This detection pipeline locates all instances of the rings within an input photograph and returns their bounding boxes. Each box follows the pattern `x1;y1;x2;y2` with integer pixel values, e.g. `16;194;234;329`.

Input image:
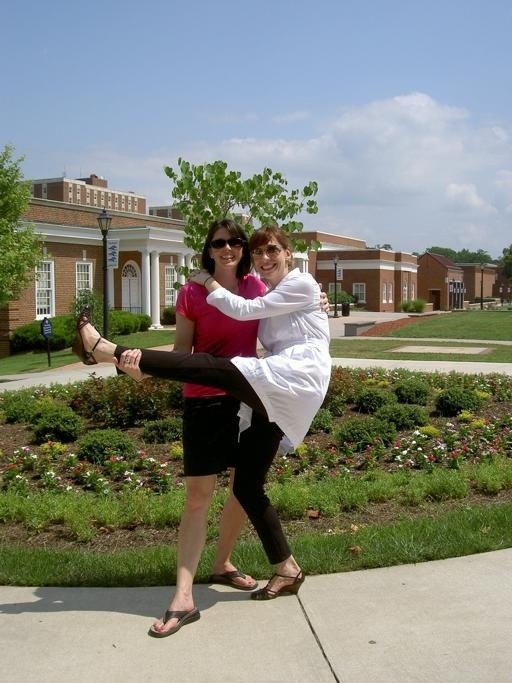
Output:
125;359;130;363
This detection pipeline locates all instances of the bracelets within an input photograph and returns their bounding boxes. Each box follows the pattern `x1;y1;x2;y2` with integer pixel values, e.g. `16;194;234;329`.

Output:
203;275;213;288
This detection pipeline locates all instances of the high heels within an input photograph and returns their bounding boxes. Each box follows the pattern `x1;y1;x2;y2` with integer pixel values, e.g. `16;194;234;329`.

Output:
148;607;200;639
250;569;306;600
211;568;259;591
71;308;103;366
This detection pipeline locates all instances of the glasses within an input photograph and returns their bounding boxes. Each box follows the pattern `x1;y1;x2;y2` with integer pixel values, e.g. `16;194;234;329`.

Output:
253;246;281;257
209;236;243;249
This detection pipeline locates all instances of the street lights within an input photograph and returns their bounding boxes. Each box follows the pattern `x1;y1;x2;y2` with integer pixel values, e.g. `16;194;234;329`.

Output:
96;206;113;340
332;254;340;318
479;261;486;311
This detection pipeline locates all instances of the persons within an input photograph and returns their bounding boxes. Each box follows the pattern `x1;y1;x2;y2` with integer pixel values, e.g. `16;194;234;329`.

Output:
72;224;333;600
113;217;330;638
